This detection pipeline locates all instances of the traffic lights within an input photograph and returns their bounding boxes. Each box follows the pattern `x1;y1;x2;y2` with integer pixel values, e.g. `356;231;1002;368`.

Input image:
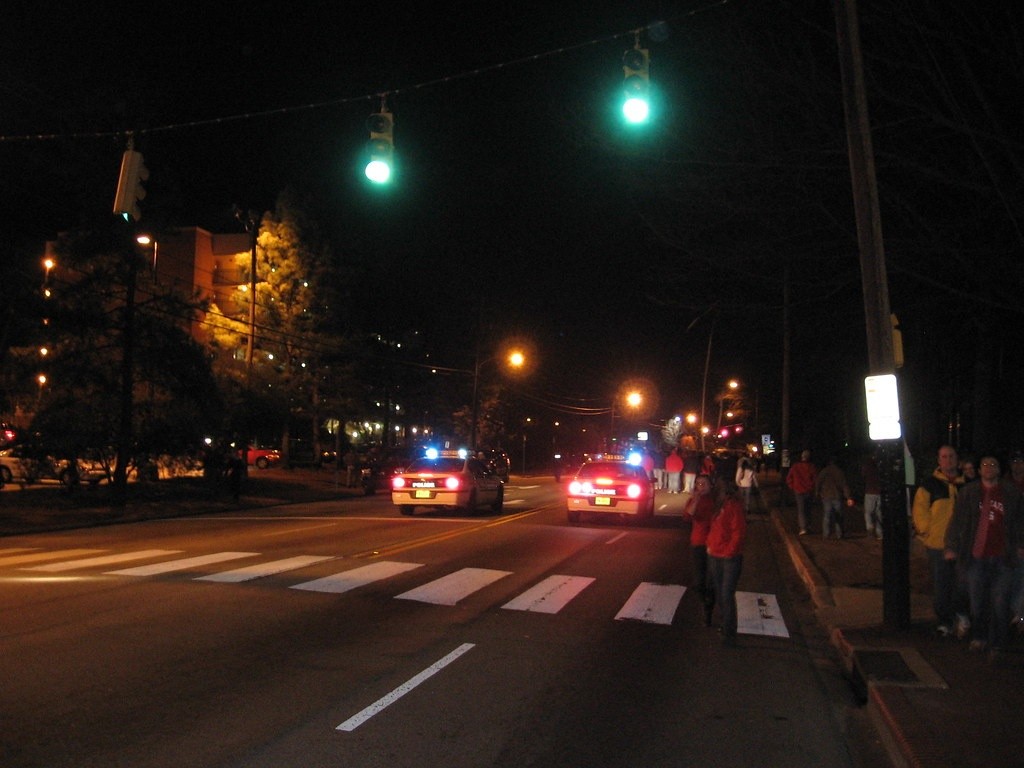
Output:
112;149;150;222
364;111;394;184
620;48;652;125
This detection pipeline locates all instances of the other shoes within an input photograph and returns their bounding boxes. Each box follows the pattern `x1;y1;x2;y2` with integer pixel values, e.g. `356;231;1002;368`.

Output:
970;637;988;653
717;628;744;647
799;528;808;536
937;624;951;636
702;610;712;626
959;615;971;637
989;646;1004;665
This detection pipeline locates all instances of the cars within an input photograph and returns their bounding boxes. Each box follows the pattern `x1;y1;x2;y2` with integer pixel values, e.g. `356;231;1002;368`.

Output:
391;450;506;518
0;445;115;486
361;450;420;495
567;460;660;524
713;448;744;474
199;441;283;469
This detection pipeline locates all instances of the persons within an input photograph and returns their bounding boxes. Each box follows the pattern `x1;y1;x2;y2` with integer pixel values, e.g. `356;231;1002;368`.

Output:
683;475;749;643
642;447;770;512
912;443;1024;663
786;450;882;539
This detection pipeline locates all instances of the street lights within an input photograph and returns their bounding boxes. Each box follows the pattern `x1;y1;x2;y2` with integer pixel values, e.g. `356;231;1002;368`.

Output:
471;346;530;451
